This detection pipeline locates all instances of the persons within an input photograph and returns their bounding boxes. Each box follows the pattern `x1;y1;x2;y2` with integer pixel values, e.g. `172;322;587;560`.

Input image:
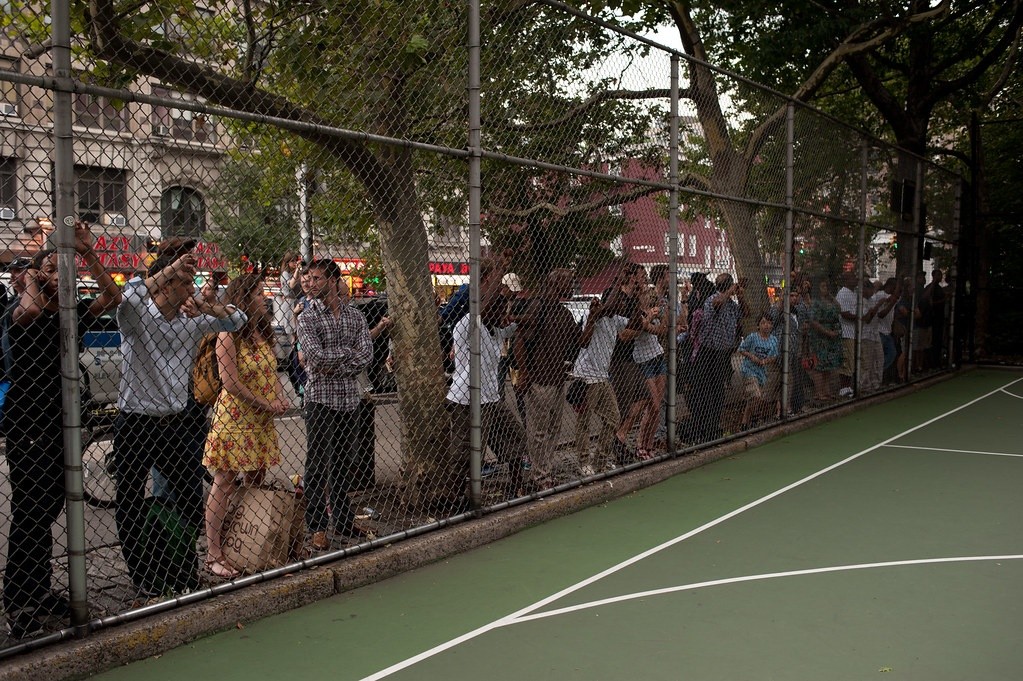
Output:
436;258;969;499
0;220;395;640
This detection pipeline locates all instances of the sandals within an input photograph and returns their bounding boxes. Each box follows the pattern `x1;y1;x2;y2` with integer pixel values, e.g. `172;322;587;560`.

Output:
204;556;241;578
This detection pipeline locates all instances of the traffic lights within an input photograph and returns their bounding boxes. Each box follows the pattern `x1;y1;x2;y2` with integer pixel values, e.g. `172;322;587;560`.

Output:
799;242;804;254
888;237;894;258
893;235;898;256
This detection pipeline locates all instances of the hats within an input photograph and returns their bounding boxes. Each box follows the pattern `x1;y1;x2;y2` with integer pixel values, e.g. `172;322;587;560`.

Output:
917;269;926;275
157;238;197;257
3;258;32;269
690;271;711;283
501;272;524;292
566;380;589;409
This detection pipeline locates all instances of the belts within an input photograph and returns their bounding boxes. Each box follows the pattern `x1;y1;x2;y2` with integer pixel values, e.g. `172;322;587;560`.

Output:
120;405;191;426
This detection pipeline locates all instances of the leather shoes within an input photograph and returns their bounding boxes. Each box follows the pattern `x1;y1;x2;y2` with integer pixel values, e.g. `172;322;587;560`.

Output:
311;531;330;549
333;526;377;538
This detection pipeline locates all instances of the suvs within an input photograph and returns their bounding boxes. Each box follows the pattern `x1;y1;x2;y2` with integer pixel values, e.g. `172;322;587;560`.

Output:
286;294;451;401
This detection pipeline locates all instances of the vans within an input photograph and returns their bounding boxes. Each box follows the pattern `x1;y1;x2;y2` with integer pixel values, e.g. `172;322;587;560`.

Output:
1;273;296;421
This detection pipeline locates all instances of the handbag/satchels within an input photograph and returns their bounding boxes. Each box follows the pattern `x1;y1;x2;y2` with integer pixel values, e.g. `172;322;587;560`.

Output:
261;485;306;559
203;475;295;574
801;333;817;370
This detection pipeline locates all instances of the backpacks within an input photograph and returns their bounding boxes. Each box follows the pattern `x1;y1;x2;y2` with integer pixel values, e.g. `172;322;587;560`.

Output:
193;331;241;405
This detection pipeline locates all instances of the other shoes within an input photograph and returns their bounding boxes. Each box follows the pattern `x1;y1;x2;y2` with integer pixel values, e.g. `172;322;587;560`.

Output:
749;413;760;428
5;606;44;637
814;393;836;400
578;465;595;476
600;462;616;472
739;423;747;431
33;592;67;615
